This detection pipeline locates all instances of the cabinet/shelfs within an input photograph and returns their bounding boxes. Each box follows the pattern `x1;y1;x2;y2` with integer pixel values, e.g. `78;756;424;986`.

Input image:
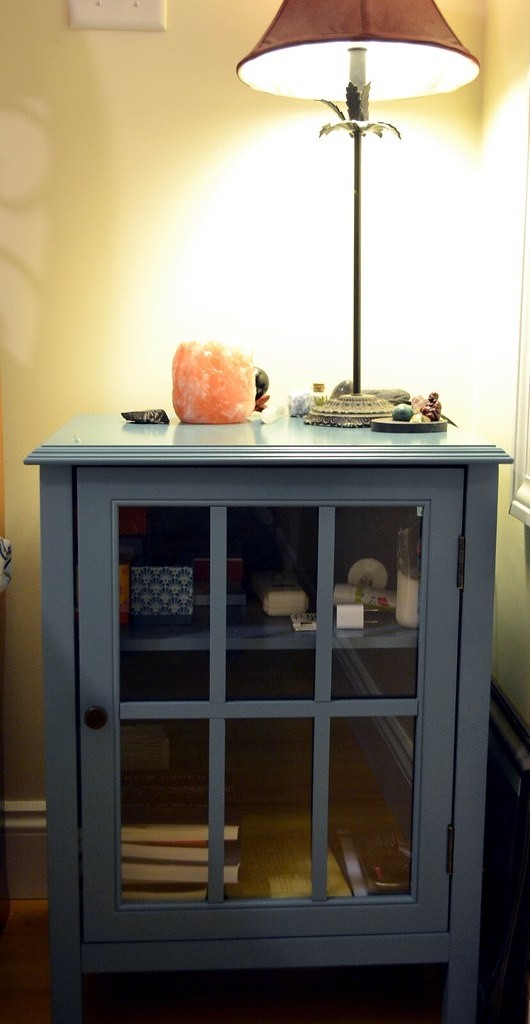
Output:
19;408;513;1023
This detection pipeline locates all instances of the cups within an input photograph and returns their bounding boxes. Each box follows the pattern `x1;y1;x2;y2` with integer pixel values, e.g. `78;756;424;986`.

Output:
395;569;419;629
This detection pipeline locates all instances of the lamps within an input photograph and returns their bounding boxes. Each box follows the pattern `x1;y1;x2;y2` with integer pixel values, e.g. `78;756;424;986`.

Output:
238;0;481;422
